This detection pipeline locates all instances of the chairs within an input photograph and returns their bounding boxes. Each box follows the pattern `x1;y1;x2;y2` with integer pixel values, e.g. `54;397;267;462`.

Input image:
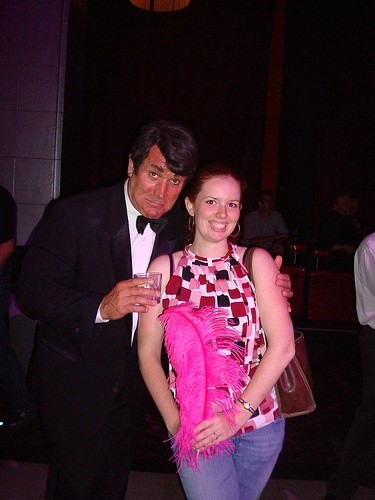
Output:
272;244;358;333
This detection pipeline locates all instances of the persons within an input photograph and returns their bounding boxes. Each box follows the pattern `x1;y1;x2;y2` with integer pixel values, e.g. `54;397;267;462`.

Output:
0;186;40;430
322;233;375;499
317;189;375;248
137;158;295;500
240;186;291;248
15;118;202;500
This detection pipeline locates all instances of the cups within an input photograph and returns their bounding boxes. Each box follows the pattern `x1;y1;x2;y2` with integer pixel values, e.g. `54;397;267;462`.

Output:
134;272;162;305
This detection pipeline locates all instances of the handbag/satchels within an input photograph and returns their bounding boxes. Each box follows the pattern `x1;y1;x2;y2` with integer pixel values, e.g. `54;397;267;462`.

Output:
243;245;318;419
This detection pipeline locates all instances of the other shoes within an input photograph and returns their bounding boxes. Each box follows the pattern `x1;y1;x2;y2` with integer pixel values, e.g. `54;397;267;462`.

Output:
0;406;40;434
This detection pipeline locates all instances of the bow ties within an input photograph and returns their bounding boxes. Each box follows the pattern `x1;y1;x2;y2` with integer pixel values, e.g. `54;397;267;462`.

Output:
135;214;168;234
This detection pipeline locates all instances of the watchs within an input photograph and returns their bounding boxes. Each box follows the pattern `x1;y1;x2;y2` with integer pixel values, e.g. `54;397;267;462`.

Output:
237;396;257;414
